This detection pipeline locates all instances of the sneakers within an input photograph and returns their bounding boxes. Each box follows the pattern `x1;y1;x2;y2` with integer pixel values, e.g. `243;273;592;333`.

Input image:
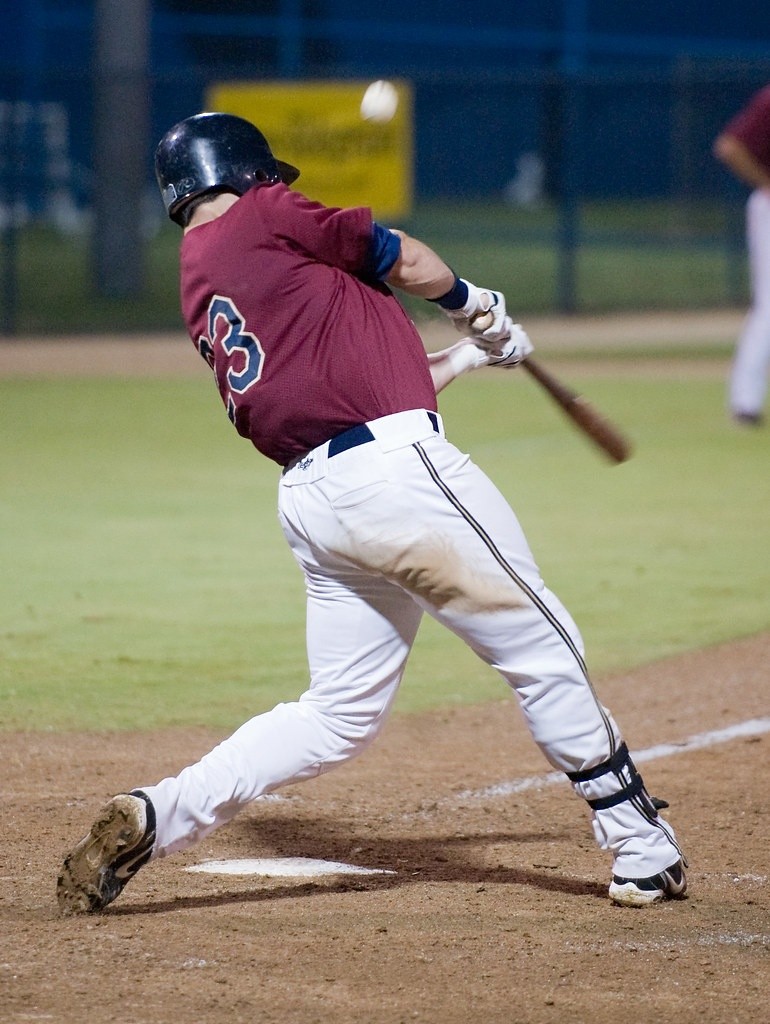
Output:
56;790;156;917
609;855;689;907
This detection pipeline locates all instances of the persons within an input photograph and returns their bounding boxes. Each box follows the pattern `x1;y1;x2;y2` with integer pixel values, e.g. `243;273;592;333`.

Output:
711;83;770;424
56;109;689;917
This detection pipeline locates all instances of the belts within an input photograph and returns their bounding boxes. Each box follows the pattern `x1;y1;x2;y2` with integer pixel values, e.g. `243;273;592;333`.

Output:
328;411;440;458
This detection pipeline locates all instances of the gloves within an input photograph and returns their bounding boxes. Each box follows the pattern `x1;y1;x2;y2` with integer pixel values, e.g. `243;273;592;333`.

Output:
437;278;512;342
458;324;534;373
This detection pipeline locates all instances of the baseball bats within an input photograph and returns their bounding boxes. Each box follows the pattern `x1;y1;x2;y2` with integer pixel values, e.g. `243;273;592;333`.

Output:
471;304;635;466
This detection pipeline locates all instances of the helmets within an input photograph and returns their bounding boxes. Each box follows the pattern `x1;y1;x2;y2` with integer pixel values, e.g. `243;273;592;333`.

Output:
154;112;300;228
